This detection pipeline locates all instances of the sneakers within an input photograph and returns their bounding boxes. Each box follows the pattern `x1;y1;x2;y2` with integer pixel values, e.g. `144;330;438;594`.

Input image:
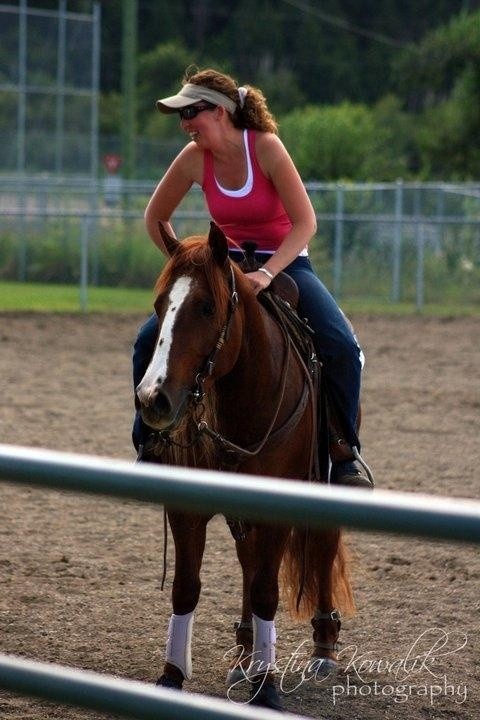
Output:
330;463;371;487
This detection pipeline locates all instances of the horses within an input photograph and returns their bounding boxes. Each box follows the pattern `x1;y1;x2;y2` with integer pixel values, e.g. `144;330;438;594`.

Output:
137;220;355;712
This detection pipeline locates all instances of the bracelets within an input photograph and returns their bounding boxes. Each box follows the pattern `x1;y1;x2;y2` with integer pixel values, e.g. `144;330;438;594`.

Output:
258;268;273;280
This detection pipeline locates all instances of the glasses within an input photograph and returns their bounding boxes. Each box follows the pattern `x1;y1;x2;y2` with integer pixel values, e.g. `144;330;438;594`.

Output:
179;106;212;120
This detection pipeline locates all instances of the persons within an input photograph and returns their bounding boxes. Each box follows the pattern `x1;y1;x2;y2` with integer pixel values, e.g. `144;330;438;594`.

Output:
132;65;373;487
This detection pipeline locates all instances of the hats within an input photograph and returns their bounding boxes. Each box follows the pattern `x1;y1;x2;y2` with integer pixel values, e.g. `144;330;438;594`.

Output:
156;83;238;115
